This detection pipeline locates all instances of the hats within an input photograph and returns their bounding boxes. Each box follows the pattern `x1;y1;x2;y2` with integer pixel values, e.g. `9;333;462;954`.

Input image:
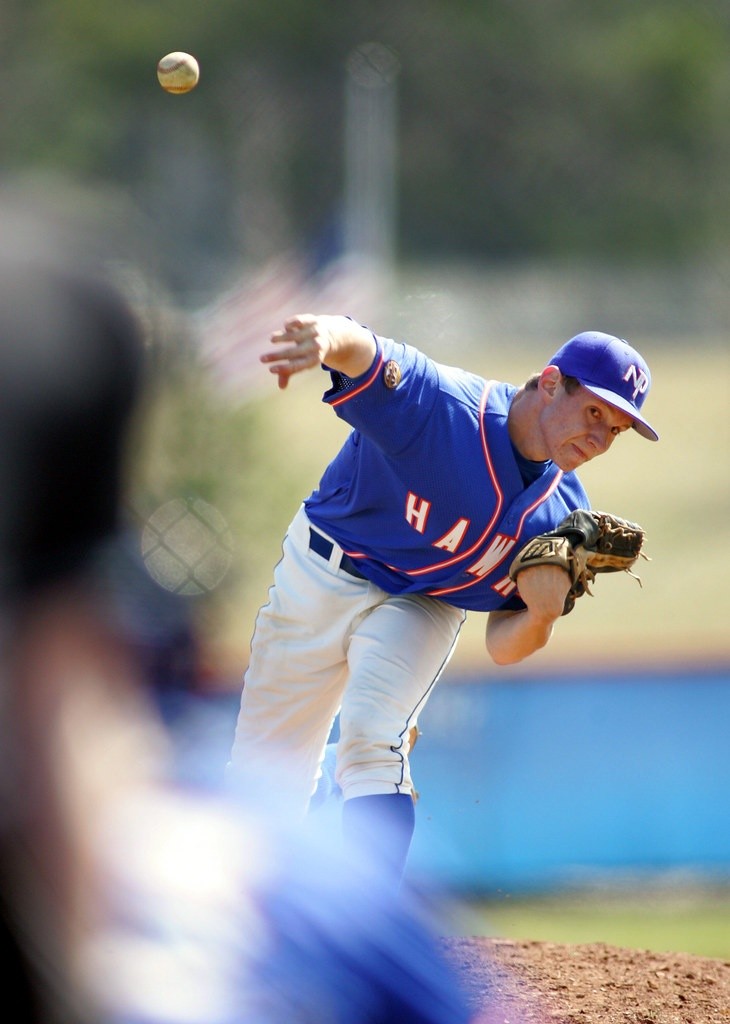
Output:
549;332;660;448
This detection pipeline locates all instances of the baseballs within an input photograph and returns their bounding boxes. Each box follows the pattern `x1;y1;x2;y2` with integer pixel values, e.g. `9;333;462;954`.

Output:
157;52;200;95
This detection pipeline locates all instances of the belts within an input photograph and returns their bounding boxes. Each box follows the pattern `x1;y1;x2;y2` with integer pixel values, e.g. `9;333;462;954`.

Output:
306;528;370;582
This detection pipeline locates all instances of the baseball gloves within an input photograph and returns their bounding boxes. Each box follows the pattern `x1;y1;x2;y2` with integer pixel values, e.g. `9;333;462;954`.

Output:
509;509;653;616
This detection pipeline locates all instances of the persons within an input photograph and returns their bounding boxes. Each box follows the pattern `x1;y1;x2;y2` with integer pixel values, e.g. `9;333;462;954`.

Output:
231;314;659;896
0;238;476;1024
